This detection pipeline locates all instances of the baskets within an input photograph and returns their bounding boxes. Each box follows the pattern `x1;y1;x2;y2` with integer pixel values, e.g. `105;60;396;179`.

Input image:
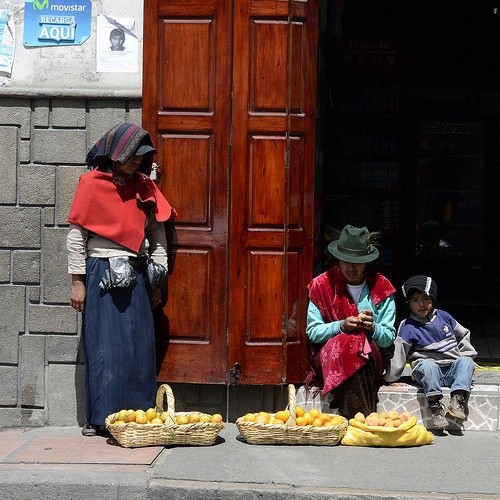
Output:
105;384;224;448
236;383;349;446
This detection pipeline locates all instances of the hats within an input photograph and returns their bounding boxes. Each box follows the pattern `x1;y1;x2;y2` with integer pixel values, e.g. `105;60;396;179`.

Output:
401;275;438;305
132;145;157;158
327;224;380;263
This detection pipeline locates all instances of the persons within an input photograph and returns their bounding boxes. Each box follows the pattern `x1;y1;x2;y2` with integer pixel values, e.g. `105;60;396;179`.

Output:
305;224;396;420
67;122;179;436
381;274;478;429
108;28;126;51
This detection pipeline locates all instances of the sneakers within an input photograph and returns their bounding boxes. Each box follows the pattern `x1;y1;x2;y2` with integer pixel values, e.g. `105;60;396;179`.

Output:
445;391;469;421
426;398;449;429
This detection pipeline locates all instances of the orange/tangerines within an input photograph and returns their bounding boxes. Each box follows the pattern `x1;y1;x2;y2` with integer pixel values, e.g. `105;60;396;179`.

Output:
111;408;223;424
243;406;345;428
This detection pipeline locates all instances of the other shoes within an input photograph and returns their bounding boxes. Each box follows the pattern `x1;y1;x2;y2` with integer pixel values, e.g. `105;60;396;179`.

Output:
82;421;100;435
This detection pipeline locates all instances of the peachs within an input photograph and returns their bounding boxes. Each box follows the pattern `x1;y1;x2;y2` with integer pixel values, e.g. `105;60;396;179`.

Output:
355;411;410;429
